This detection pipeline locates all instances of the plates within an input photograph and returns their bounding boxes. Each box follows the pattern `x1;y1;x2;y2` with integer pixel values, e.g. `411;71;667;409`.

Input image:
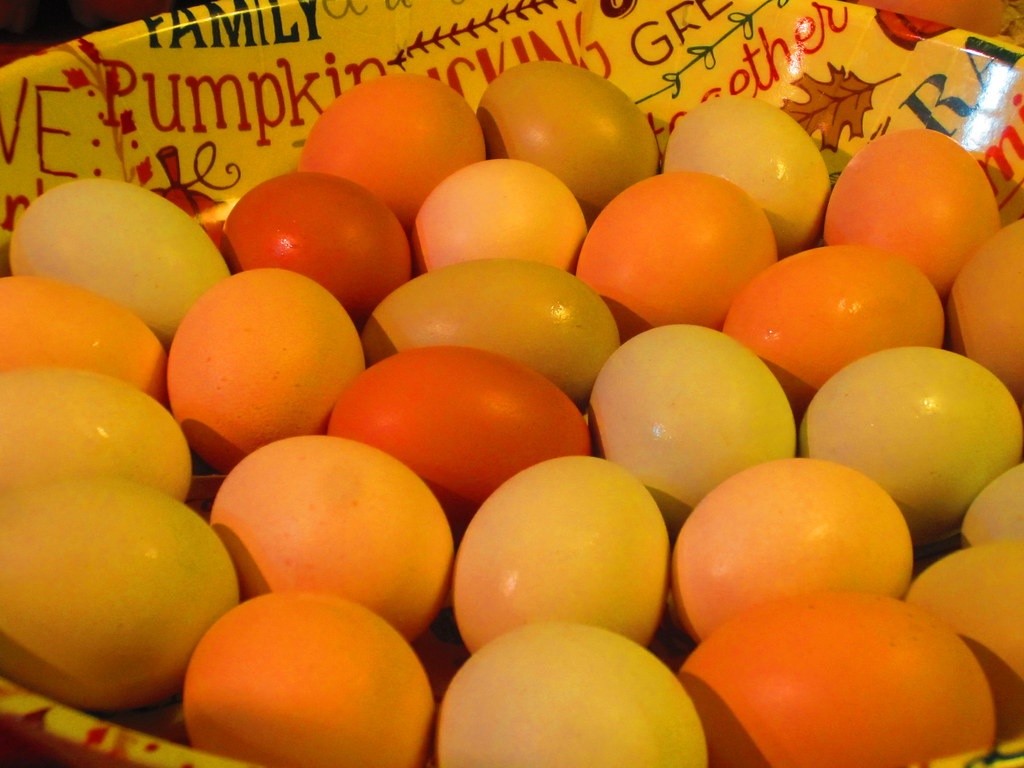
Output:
0;0;1024;768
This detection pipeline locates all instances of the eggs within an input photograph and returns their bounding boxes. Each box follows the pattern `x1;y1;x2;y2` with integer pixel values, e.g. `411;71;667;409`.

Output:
0;58;1022;768
